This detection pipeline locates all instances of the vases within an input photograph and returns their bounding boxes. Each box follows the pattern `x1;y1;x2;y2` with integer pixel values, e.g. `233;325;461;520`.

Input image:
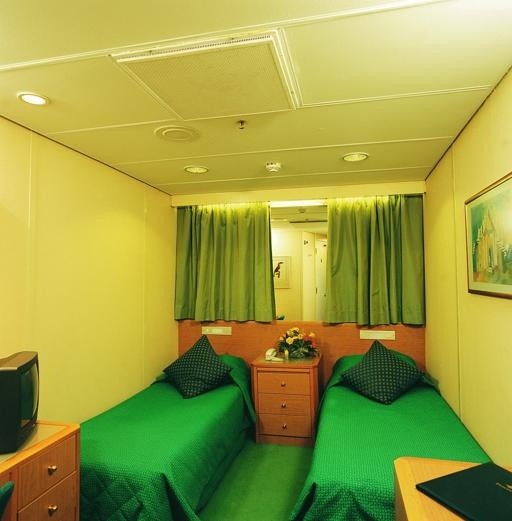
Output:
287;350;304;358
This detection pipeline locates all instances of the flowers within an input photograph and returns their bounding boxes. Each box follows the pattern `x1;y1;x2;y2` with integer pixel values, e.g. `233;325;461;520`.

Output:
274;327;320;360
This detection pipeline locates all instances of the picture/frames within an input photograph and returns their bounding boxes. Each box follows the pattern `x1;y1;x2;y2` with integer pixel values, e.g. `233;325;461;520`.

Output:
462;171;512;301
272;255;290;289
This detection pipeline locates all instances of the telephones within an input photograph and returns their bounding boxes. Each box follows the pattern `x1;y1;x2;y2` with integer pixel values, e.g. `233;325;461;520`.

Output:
265;347;277;361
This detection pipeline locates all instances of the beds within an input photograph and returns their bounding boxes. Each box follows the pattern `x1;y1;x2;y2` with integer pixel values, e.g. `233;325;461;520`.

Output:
285;348;493;521
80;353;257;521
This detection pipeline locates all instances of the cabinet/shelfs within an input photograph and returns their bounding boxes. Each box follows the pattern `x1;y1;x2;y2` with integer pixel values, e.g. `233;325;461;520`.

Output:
391;454;512;521
0;418;82;521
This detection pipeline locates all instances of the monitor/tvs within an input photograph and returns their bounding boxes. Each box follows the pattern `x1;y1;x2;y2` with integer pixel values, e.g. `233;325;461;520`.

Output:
0;350;41;454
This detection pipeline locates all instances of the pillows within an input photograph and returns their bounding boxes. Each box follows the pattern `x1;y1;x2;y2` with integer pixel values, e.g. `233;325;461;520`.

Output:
339;339;425;406
161;334;234;400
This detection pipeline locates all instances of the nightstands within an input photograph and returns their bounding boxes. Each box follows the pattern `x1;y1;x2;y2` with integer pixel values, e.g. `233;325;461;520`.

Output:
249;352;325;449
